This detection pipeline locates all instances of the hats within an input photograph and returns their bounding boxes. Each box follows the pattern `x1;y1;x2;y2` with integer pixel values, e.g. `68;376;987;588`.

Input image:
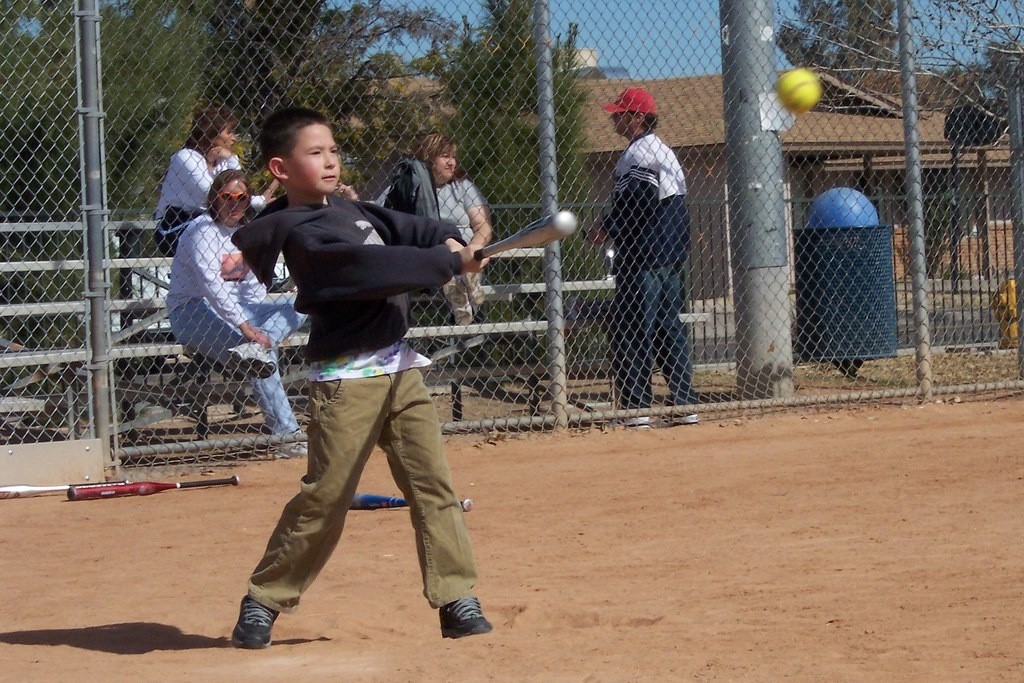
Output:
605;87;656;113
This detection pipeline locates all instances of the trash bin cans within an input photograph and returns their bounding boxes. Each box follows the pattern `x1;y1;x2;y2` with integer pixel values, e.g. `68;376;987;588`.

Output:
791;222;901;376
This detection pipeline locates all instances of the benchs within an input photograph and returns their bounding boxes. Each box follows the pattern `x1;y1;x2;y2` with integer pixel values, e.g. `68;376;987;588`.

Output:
131;247;710;441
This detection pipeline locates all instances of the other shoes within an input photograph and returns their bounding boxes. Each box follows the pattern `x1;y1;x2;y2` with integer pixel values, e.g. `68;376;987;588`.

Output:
610;412;650;427
461;271;484;304
442;276;472;325
662;410;699;423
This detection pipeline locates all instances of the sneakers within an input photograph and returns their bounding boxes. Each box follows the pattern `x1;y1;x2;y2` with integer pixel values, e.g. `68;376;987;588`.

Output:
439;594;492;639
227;341;276;379
231;594;281;649
273;429;309;459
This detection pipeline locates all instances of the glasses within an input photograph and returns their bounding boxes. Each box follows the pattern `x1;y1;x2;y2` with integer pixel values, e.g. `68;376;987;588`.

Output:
218;192;249;204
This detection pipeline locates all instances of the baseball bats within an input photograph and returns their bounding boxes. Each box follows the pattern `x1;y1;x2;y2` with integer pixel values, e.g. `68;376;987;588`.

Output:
349;492;473;511
68;475;240;501
0;480;134;499
474;210;578;261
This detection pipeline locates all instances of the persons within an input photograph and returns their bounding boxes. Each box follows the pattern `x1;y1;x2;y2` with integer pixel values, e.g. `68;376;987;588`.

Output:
231;108;493;652
151;101;493;458
587;86;702;430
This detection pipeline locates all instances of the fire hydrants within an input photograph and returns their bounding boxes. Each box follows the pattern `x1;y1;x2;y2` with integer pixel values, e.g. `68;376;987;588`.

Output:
991;277;1019;349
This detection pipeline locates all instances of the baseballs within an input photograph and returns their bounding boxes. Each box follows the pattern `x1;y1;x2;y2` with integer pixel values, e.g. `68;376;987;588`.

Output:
776;68;822;111
